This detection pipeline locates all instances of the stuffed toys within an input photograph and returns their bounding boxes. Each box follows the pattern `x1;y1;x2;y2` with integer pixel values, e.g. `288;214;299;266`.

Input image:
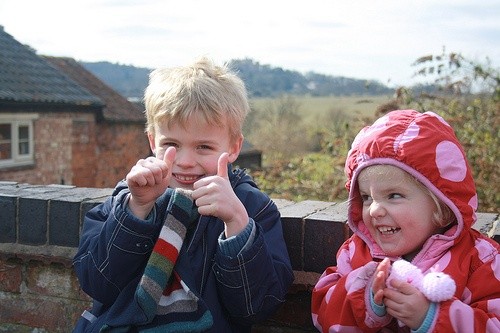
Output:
386;259;456;328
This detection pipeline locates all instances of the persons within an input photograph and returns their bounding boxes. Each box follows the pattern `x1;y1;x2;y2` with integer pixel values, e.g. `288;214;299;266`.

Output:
311;109;500;333
71;55;295;333
